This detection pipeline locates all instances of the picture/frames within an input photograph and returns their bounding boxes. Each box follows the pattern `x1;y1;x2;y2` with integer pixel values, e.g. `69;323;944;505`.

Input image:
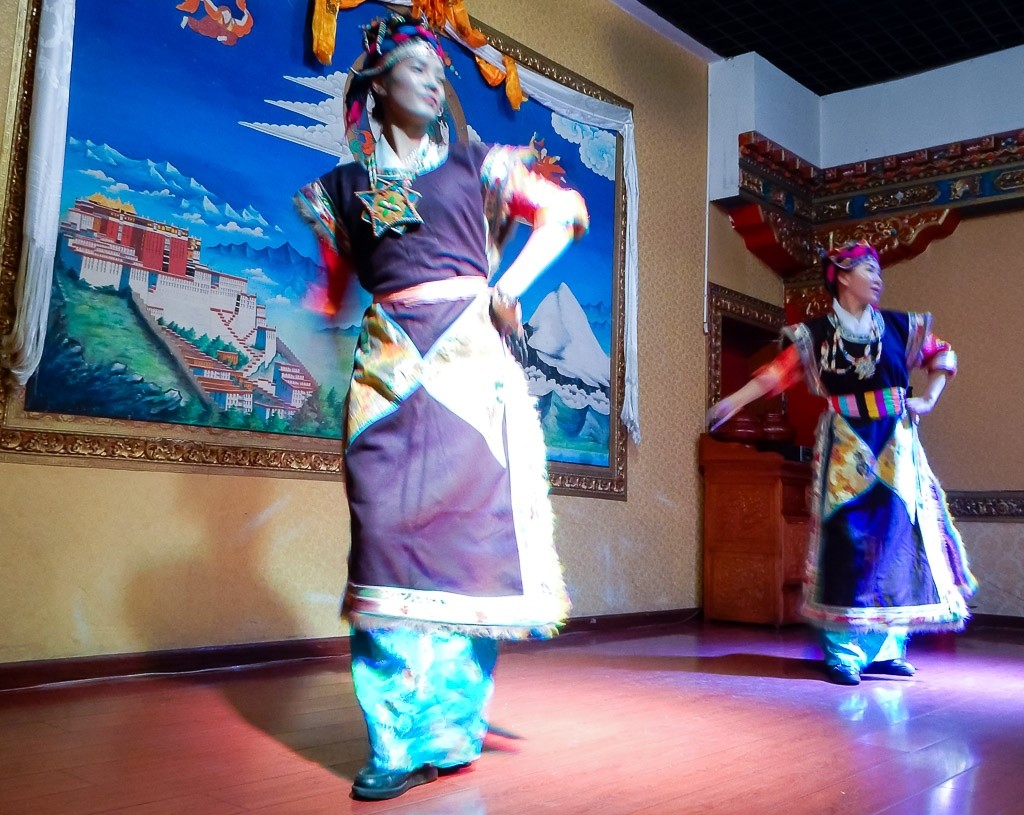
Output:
0;0;635;499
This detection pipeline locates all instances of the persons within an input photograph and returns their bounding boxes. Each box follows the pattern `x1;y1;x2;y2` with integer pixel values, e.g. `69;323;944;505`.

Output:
289;22;594;804
703;235;978;684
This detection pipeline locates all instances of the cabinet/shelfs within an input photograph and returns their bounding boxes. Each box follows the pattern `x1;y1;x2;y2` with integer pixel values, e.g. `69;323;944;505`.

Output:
699;433;810;627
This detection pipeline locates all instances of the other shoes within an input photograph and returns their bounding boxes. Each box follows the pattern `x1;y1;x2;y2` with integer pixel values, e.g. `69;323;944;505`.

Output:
871;660;916;676
351;762;438;798
827;664;860;685
